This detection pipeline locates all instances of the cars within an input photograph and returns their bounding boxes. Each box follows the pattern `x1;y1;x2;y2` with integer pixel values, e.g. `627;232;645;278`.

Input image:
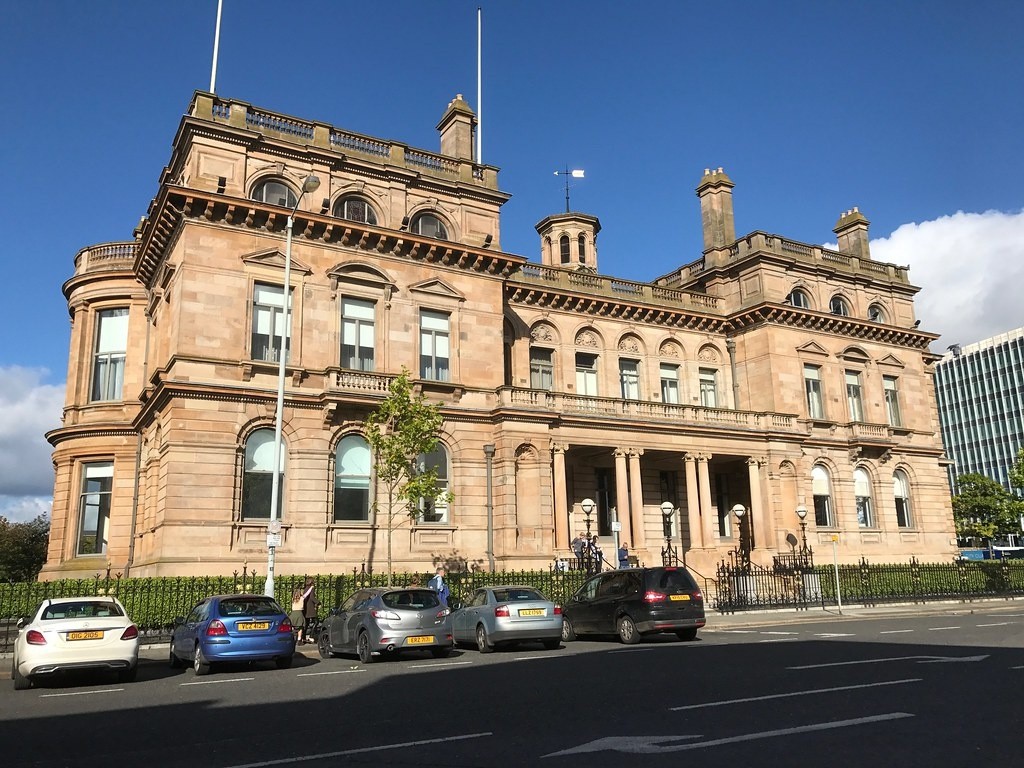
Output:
170;594;299;676
449;584;566;653
317;586;455;664
9;595;139;690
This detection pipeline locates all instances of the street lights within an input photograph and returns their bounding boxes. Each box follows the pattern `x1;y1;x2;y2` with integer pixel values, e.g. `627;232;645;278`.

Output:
265;177;333;598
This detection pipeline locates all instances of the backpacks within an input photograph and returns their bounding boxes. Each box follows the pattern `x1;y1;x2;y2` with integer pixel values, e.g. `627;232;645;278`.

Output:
427;576;438;593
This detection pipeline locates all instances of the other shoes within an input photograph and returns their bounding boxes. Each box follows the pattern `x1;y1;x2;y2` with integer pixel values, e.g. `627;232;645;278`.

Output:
297;639;311;646
306;636;315;642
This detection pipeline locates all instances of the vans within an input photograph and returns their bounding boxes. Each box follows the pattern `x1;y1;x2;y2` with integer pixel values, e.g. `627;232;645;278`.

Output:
555;567;708;644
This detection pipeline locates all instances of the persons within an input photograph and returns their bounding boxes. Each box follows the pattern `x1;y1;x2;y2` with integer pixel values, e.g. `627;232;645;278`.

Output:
571;532;586;570
290;585;315;646
618;542;630;569
301;579;321;644
588;535;606;575
428;567;450;607
